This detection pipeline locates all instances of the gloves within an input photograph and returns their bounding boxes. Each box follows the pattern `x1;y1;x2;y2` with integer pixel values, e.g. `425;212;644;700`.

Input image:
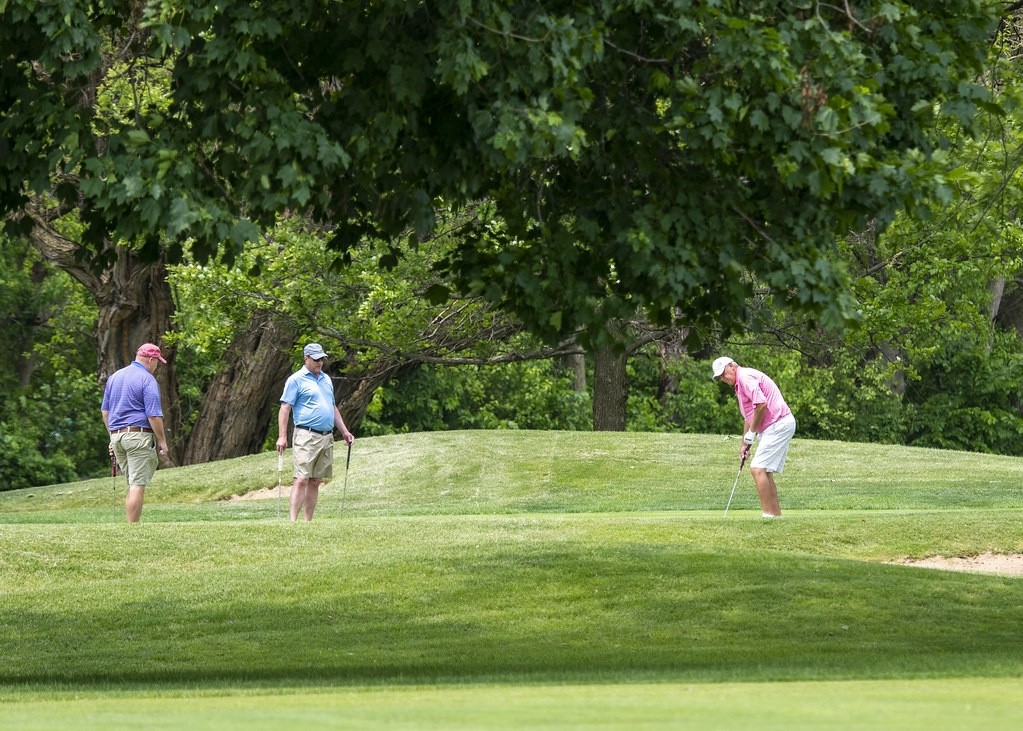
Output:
744;430;756;444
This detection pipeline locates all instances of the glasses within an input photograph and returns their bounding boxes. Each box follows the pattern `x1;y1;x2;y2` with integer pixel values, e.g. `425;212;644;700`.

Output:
313;357;324;362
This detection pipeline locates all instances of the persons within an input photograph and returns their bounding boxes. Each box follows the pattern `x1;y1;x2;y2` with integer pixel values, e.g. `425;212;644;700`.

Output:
276;343;354;522
100;344;168;523
713;356;795;517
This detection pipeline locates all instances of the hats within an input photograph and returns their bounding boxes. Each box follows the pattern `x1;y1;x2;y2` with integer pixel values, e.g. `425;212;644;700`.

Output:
137;343;167;364
712;357;733;381
304;343;328;360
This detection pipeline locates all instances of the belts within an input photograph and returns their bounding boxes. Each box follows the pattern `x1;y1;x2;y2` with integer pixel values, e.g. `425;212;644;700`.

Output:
111;426;154;433
295;425;334;436
